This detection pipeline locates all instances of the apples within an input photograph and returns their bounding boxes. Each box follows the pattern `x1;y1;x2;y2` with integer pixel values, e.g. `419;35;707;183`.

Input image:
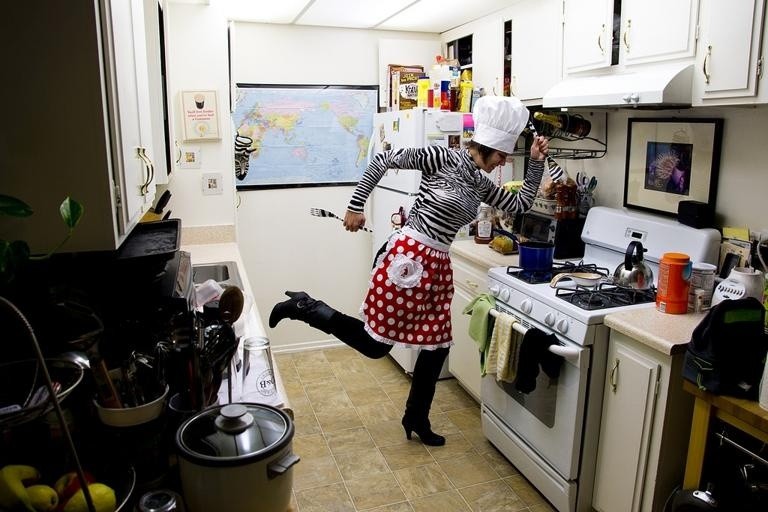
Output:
53;472;95;512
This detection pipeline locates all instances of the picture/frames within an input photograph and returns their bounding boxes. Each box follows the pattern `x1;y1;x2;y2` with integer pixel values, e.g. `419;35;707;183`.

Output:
202;173;223;195
180;142;205;169
623;118;724;217
182;90;220;139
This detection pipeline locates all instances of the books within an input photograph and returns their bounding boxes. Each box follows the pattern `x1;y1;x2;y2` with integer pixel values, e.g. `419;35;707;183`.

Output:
385;63;426;112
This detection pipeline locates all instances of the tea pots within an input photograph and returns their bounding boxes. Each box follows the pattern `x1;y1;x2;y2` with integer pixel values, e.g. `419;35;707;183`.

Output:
611;239;654;290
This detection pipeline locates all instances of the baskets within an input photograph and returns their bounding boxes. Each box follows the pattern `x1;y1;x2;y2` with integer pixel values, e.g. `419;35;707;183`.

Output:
529;197;561;216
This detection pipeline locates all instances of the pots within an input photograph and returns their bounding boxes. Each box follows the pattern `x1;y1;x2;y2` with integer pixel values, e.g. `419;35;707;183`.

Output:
492;225;557;276
170;399;302;511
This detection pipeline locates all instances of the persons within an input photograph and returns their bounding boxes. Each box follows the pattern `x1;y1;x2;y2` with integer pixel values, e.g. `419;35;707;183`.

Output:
268;134;550;446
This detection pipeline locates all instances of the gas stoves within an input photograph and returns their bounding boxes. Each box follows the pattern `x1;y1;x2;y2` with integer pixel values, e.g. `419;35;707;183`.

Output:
486;255;656;346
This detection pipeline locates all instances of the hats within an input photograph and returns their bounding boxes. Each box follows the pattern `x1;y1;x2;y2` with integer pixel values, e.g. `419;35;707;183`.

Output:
471;94;530;154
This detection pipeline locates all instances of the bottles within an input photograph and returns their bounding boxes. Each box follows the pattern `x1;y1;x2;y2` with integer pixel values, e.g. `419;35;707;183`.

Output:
473;208;496;243
656;252;691;314
416;76;430;106
532;111;592;138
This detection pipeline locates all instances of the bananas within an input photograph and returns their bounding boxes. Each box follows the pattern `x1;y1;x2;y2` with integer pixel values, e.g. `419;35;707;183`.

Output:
0;465;41;512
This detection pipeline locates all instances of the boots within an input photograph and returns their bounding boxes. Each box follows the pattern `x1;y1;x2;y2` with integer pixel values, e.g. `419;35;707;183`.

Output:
402;385;445;447
268;290;338;336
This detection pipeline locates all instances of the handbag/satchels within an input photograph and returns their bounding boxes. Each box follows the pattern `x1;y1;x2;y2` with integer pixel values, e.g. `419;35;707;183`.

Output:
682;298;766;400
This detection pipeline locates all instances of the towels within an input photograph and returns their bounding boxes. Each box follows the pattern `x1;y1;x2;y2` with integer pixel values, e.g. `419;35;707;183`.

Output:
462;292;560;393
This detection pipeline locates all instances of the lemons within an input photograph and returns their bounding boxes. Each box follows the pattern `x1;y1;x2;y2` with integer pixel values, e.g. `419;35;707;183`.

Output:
63;483;116;512
25;485;59;512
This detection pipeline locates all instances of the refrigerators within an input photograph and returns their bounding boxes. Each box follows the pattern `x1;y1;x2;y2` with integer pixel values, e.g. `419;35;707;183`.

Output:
366;108;525;382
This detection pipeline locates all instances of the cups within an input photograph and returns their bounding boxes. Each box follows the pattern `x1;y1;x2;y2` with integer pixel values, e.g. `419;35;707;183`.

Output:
574;192;595;218
139;489;180;512
552;184;579;218
242;336;277;405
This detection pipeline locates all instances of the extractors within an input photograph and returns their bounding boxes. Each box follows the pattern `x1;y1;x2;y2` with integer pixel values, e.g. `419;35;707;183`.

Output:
541;63;695;111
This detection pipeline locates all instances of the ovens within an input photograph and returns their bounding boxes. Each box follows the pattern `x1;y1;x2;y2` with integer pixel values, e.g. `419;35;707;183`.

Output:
478;298;609;512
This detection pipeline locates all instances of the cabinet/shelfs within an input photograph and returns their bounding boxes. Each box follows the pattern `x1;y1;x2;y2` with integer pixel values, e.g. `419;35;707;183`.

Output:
563;0;700;72
591;329;671;512
448;261;488;405
0;0;155;253
440;3;543;107
143;1;182;185
691;0;768;107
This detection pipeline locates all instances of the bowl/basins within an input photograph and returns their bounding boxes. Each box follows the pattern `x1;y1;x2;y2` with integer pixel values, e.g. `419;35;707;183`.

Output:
90;387;169;425
